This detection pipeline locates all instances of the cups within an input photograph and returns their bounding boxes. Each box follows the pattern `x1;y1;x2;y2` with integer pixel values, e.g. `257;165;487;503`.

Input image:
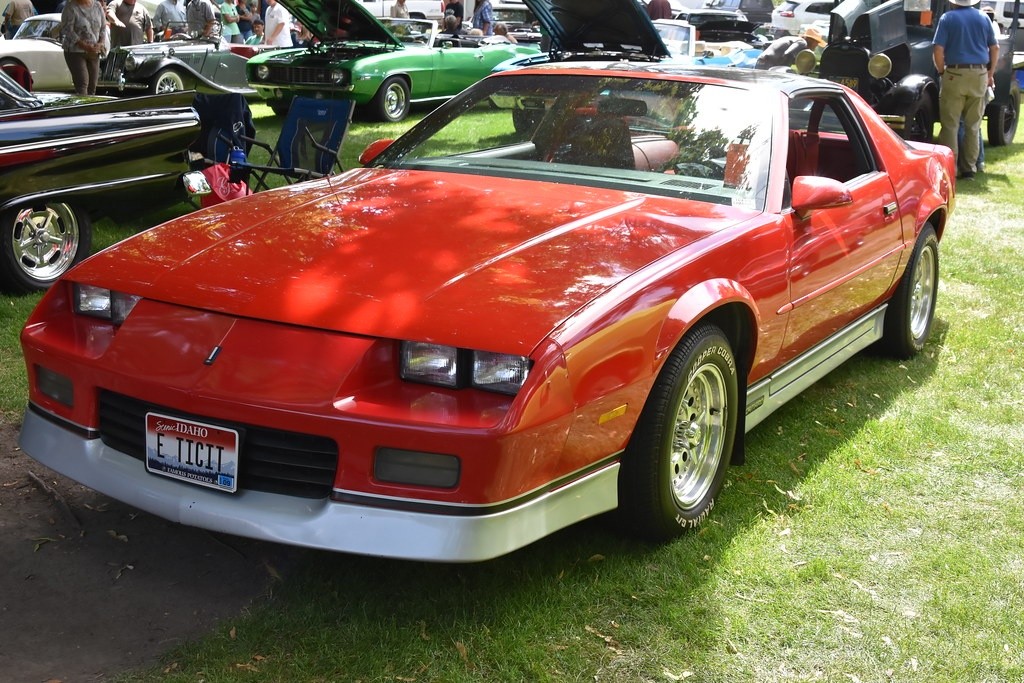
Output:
986;85;995;101
191;31;198;38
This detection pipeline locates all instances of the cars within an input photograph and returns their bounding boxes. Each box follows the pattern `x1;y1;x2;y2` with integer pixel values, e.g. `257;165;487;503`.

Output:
14;56;957;566
2;0;1023;150
0;64;204;295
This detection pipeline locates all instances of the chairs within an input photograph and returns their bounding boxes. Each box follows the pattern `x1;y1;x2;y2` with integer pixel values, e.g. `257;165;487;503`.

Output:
787;131;821;186
172;87;360;211
438;37;462;48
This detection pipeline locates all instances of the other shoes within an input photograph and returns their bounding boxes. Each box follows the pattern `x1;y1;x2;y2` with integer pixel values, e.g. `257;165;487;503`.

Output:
961;172;974;178
976;162;985;172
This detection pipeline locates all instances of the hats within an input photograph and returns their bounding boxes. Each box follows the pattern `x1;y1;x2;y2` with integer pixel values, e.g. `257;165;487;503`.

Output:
949;0;980;6
798;26;828;48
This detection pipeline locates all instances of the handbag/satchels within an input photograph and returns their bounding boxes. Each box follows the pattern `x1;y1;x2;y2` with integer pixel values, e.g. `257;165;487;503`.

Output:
199;164;253;209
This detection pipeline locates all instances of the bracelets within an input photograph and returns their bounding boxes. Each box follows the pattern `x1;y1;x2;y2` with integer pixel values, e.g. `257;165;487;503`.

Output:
938;72;943;75
147;41;153;43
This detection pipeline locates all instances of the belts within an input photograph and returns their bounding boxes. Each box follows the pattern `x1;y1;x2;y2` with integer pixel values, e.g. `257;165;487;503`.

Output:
948;64;987;68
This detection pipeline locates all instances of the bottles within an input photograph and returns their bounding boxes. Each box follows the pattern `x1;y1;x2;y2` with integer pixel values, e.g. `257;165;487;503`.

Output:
164;29;172;39
231;146;245;163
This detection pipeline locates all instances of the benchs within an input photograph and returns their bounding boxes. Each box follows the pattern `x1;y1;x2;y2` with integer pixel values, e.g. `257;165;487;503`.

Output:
425;35;476;48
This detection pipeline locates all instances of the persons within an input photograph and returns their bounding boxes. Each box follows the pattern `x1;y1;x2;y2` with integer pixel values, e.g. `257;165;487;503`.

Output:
473;0;493;36
61;0;106;95
442;0;463;31
99;0;154;48
755;28;827;70
929;0;985;172
932;0;999;179
221;0;313;48
1;0;66;39
151;0;188;33
982;5;1000;34
494;22;518;43
470;28;482;36
439;15;468;35
646;0;672;20
186;0;215;39
390;0;413;35
